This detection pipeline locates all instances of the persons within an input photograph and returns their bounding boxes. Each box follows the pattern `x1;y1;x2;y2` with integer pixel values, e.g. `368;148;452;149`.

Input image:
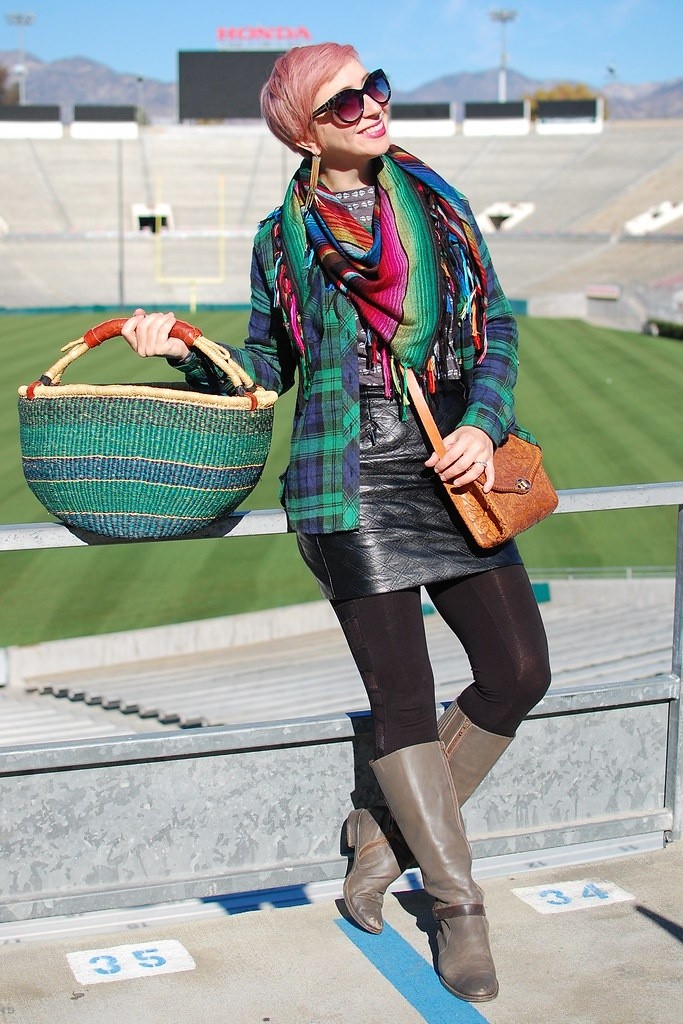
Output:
117;42;551;1001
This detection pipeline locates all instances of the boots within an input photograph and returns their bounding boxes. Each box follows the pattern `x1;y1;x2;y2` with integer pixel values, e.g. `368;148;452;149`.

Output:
343;699;516;933
369;741;499;1002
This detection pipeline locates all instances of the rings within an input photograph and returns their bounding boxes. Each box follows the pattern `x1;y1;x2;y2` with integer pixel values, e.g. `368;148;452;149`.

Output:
474;461;487;467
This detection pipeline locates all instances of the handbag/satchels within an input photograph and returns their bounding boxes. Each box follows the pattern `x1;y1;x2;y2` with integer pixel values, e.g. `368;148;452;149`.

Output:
439;433;558;549
18;318;277;538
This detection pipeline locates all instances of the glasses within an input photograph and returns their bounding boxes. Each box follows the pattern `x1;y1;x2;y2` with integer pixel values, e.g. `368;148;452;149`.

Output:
312;70;390;123
